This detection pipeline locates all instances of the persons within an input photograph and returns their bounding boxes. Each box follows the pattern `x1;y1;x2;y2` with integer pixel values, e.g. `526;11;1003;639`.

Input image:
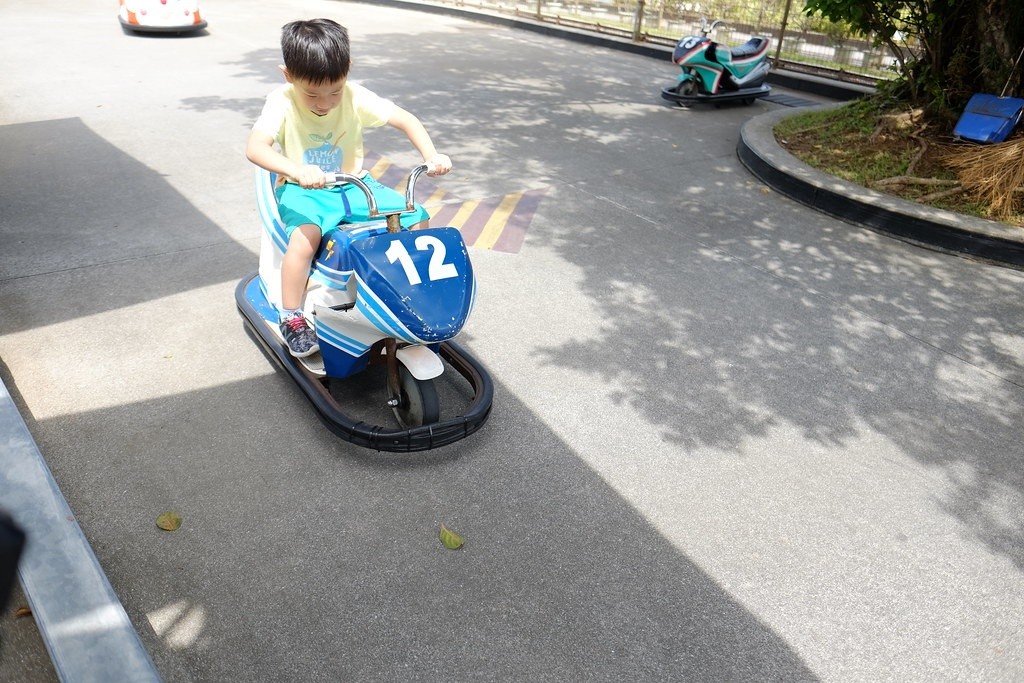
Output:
246;19;453;358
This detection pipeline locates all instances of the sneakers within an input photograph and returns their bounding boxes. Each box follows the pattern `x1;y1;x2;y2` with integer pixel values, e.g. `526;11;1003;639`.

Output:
278;310;320;358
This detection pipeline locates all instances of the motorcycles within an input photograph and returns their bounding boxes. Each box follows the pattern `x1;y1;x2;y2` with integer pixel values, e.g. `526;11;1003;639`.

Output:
660;16;772;108
235;152;493;453
